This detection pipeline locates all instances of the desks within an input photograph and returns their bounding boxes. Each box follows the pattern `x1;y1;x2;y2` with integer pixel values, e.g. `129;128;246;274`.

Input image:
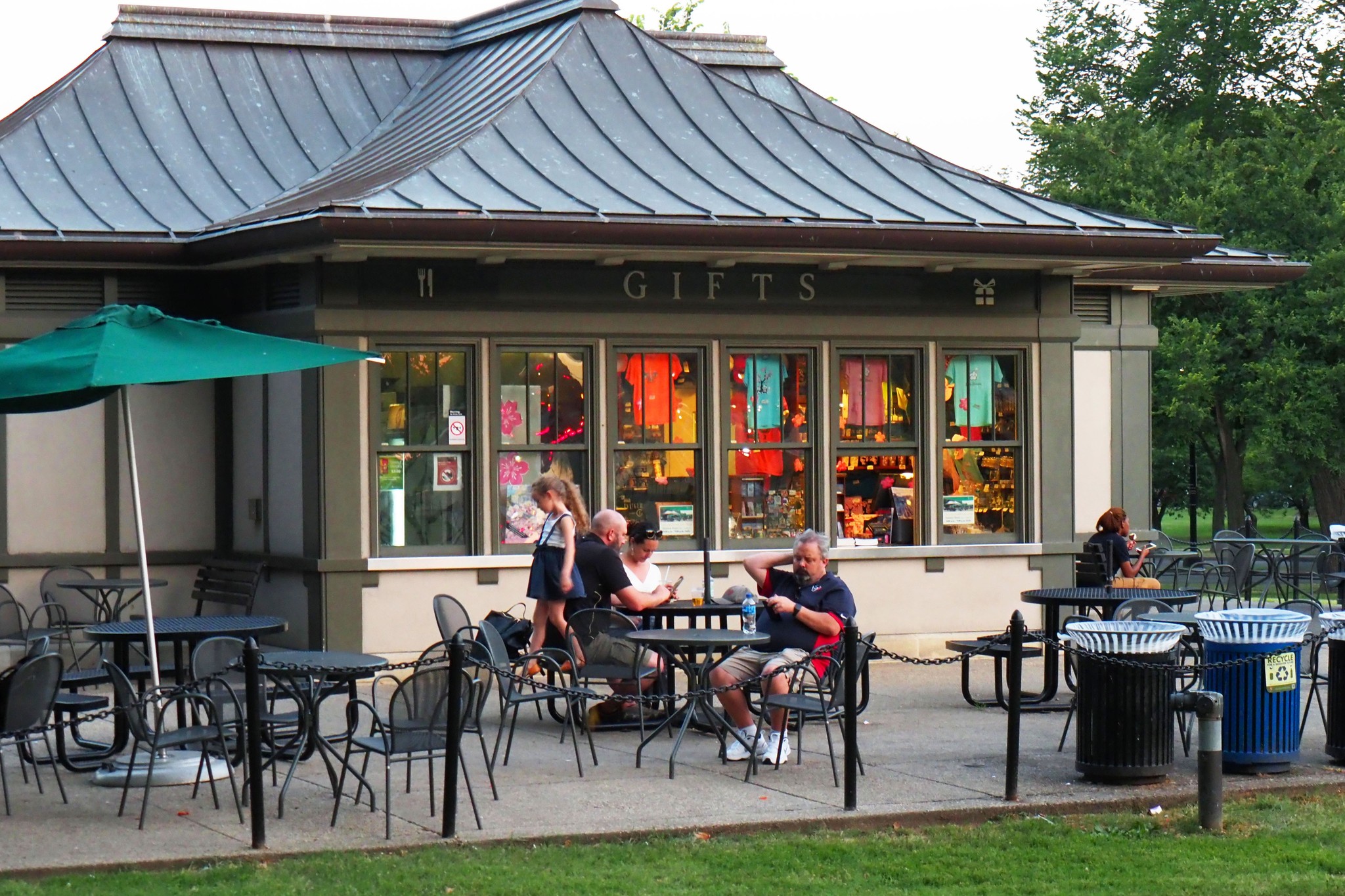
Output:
1214;538;1335;615
1020;587;1198;710
621;629;774;779
82;616;288;757
1130;551;1198;613
227;650;389;819
56;578;169;668
616;595;771;727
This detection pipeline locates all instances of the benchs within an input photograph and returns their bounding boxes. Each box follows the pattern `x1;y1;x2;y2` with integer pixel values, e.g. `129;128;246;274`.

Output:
189;562;264;665
946;628;1062;658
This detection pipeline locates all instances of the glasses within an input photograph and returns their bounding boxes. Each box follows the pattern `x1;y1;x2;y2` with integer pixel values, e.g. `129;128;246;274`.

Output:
636;529;663;539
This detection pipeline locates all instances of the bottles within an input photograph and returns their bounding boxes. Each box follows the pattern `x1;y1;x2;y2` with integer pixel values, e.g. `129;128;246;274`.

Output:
741;593;756;633
702;570;714;597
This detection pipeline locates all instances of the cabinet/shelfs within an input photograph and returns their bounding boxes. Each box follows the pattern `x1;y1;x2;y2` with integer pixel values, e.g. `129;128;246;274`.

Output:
732;472;769;539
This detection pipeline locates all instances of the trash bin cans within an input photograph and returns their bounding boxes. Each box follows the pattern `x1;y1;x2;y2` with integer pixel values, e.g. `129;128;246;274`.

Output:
1065;621;1187;785
1193;608;1312;775
1318;611;1345;764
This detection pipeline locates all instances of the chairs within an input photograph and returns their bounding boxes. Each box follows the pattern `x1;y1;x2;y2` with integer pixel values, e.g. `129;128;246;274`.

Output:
1056;531;1345;758
0;566;880;839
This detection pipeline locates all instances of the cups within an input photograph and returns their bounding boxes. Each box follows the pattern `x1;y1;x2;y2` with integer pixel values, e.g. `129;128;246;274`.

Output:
690;589;704;606
659;580;673;605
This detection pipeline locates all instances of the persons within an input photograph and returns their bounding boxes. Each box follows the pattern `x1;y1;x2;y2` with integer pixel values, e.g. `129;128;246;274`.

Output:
542;509;670;719
710;528;857;765
608;522;679;671
1087;508;1160;614
513;475;591;684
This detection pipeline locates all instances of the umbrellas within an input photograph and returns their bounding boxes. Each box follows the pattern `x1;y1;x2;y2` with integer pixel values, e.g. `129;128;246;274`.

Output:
0;302;385;760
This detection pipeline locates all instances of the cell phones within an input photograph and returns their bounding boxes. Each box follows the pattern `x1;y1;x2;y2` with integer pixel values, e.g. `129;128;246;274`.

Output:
1136;542;1156;551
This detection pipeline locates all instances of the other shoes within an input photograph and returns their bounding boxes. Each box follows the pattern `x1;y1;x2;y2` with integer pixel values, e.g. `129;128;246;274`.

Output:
514;664;540;683
588;702;666;725
561;658;585;670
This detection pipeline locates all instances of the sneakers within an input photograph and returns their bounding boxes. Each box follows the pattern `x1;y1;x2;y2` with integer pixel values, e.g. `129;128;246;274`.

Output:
726;729;768;760
762;733;790;765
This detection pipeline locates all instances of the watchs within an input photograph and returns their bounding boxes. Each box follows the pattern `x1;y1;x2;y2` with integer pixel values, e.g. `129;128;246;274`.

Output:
792;604;801;617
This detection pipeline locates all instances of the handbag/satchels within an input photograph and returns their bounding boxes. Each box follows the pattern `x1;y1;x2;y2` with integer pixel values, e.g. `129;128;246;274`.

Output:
469;602;533;660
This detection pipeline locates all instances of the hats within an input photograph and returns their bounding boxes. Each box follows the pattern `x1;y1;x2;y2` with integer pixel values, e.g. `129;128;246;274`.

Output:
710;585;759;605
